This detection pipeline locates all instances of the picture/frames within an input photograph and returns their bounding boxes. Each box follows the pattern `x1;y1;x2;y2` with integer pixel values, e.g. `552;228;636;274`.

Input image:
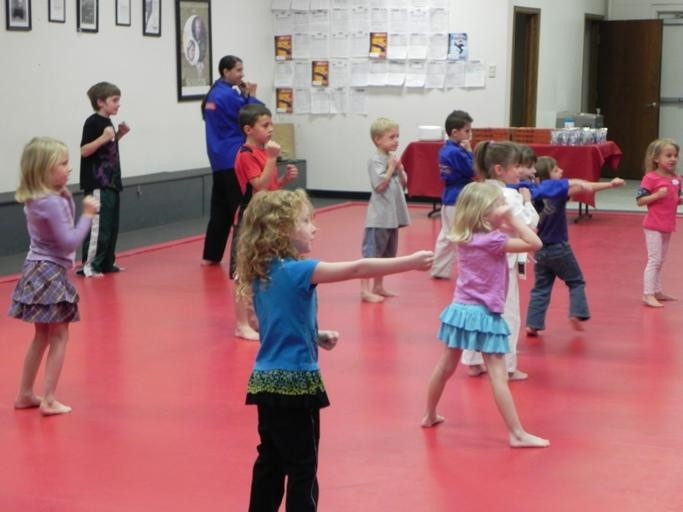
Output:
142;0;161;36
7;0;31;31
77;0;98;32
49;0;65;23
175;0;212;102
116;0;131;25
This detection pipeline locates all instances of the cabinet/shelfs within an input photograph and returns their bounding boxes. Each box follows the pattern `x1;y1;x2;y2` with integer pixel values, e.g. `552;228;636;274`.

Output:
0;159;306;277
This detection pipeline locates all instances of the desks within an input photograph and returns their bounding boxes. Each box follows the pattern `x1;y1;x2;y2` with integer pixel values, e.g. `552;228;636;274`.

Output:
408;141;614;225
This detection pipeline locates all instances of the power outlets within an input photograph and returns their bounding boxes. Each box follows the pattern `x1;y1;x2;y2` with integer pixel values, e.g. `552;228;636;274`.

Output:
489;66;496;77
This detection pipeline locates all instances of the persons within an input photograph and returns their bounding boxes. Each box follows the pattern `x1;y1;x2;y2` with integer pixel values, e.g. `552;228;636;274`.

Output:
80;82;130;278
10;137;101;416
191;18;205;61
635;139;682;307
432;110;476;280
201;55;264;267
360;119;408;303
233;189;435;511
504;141;592;211
419;181;549;448
462;140;539;382
526;155;625;338
229;102;299;340
